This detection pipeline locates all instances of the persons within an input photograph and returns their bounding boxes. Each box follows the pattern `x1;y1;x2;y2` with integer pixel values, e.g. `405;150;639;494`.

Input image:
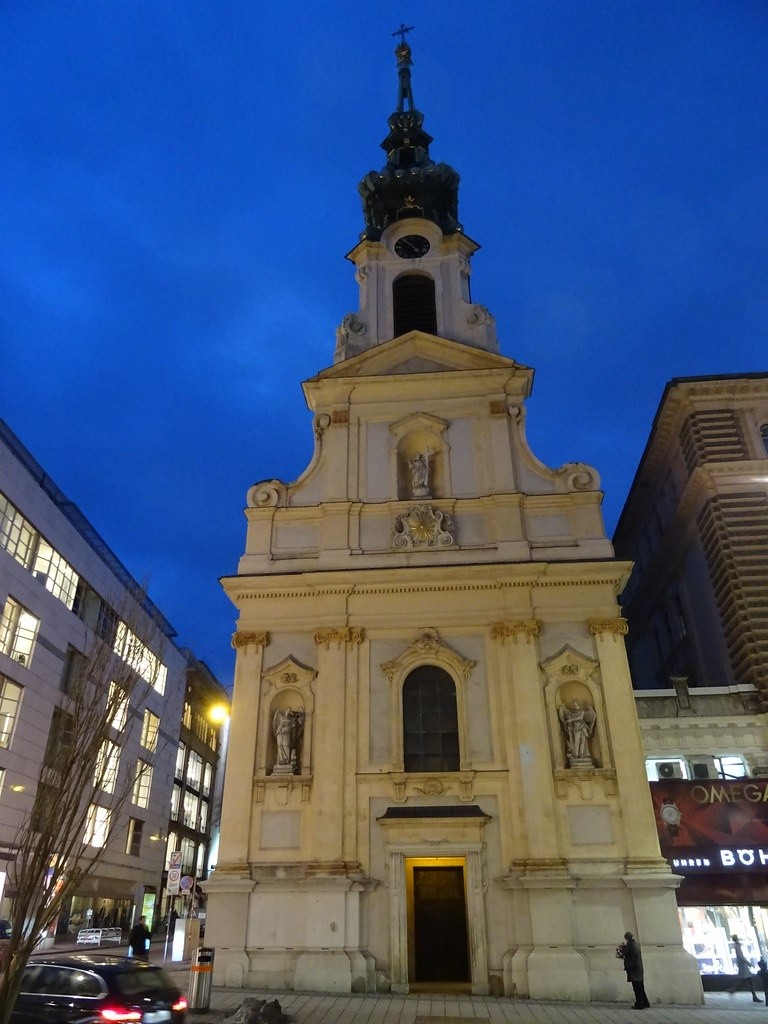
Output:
275;706;302;765
564;697;593;759
728;934;763;1003
129;916;153;962
406;452;434;496
93;909;179;932
623;932;650;1010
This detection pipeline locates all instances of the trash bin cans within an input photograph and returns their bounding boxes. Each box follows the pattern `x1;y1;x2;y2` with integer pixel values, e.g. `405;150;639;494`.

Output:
187;947;216;1014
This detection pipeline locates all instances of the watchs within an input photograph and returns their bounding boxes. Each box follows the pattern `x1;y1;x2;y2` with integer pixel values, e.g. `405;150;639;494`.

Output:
660;798;683;838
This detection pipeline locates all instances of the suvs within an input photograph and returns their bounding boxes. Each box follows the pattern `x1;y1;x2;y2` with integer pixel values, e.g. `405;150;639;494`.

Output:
0;954;188;1024
